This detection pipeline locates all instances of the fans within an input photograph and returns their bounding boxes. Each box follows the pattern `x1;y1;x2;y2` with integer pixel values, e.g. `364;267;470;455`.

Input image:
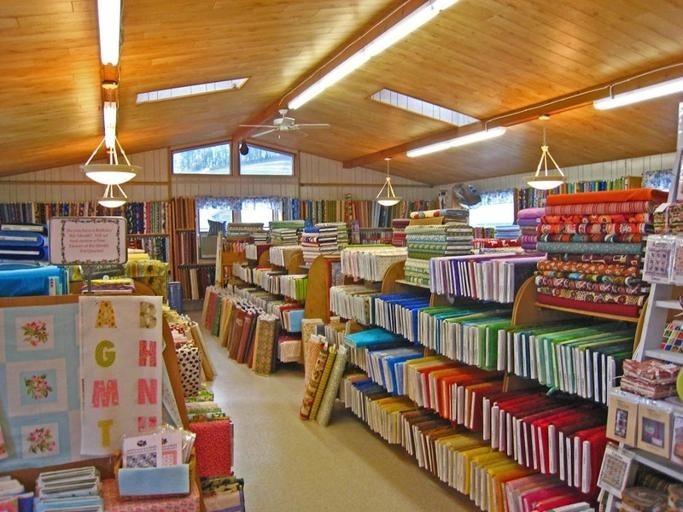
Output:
236;109;332;139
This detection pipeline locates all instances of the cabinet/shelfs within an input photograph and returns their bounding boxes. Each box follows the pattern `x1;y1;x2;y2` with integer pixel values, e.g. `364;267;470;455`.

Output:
1;222;242;512
214;228;683;511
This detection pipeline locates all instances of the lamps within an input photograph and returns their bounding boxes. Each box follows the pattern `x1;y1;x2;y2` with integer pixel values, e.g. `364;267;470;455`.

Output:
79;129;141;209
520;114;567;193
372;156;403;207
